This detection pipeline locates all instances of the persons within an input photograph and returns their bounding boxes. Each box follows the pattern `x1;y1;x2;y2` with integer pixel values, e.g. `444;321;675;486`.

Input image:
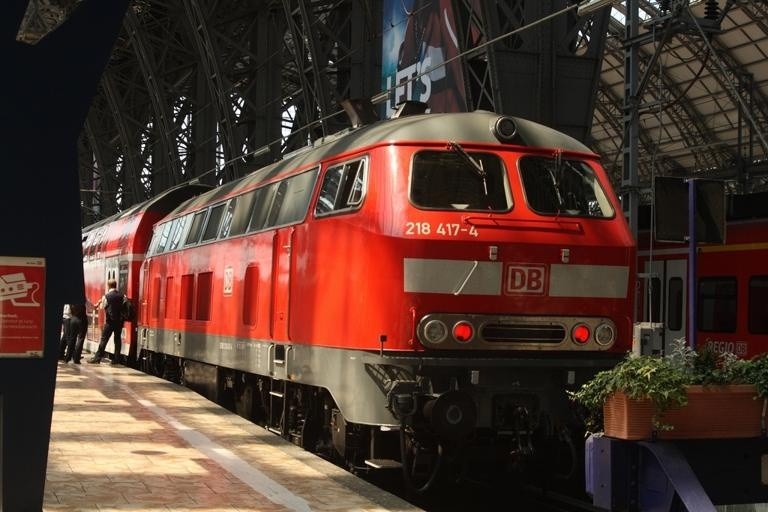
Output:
64;298;102;363
87;278;130;365
55;303;84;361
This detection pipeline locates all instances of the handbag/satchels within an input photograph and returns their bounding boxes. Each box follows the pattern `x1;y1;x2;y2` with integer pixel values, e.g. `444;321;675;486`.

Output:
119;300;135;320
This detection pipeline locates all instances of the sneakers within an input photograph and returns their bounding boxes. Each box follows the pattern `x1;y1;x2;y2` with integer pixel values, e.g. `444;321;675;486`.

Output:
57;355;84;364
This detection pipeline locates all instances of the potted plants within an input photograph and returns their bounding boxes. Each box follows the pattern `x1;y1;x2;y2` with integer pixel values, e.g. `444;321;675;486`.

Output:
564;336;768;443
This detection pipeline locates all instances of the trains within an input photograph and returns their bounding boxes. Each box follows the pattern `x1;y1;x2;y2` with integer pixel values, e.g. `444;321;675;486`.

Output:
61;109;639;498
632;214;768;381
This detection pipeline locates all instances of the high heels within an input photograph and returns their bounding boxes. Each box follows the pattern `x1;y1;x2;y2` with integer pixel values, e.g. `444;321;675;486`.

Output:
87;359;101;363
